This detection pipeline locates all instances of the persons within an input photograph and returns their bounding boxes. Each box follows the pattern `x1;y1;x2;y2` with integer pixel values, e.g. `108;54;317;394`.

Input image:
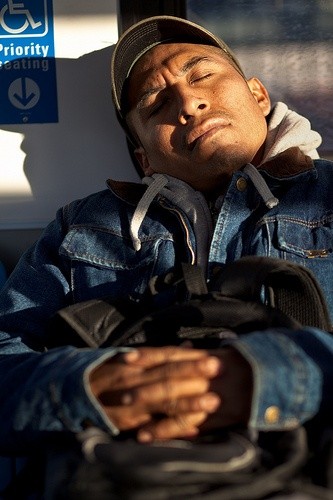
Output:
0;16;333;500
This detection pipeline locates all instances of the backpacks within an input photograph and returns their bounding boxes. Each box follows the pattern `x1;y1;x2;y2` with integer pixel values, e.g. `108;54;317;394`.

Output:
60;261;333;500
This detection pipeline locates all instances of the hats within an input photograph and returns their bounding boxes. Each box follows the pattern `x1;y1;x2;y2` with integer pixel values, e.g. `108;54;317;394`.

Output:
111;17;244;145
